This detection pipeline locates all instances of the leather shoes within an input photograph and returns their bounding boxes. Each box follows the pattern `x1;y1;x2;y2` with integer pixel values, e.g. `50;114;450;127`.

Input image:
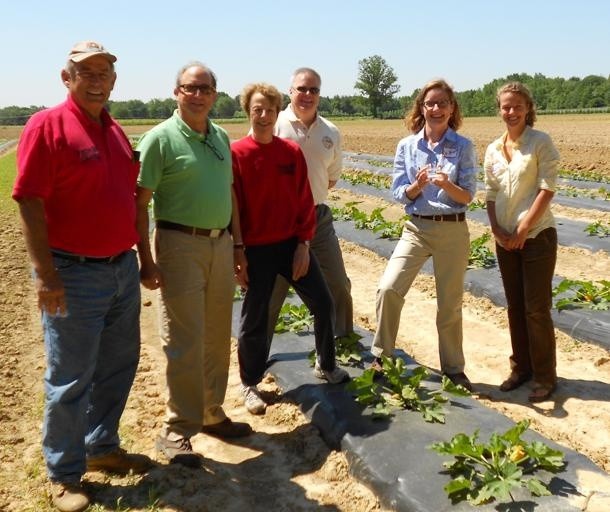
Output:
155;433;204;468
452;373;472;390
84;450;152;473
201;417;251;438
51;479;89;512
369;357;384;373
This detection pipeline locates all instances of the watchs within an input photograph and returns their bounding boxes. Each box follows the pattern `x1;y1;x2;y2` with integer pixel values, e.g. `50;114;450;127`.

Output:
299;240;311;248
233;245;246;251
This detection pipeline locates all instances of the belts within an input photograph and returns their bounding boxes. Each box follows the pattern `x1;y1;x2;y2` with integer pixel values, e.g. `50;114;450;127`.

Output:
416;213;466;222
157;222;226;240
46;250;127;267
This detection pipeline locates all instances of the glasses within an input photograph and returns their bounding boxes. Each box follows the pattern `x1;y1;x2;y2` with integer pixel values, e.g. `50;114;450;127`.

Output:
295;85;320;94
201;140;224;161
423;98;450;108
178;82;214;92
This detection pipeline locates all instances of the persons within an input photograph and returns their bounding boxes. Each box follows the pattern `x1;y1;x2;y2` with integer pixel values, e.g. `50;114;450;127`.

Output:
134;63;253;469
368;233;370;234
369;79;475;392
483;84;560;402
228;82;348;415
12;42;153;511
246;68;364;354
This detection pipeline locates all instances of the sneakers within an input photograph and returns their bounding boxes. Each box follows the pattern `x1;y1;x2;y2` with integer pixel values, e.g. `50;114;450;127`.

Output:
240;384;266;414
314;358;349;384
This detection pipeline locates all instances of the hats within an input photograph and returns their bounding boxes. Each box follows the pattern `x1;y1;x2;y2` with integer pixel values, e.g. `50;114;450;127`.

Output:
66;41;117;64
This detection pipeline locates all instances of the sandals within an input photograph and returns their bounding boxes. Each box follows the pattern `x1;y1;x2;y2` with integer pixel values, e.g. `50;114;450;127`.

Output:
529;382;557;400
499;377;520;390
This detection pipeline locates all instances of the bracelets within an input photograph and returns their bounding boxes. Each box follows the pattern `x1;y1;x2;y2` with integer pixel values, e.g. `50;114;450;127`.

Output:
234;243;243;245
